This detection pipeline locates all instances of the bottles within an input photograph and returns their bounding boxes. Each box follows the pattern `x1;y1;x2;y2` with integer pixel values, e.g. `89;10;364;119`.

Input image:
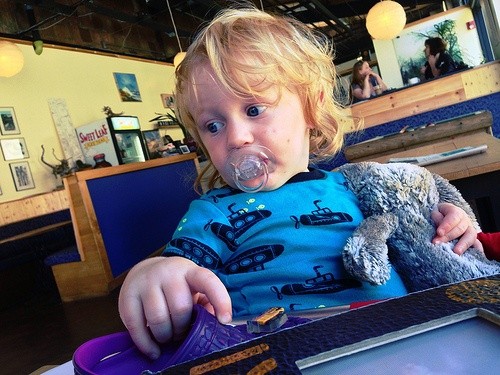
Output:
93;153;113;169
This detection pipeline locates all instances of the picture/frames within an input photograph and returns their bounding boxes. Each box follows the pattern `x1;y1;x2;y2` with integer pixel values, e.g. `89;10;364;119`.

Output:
0;136;31;161
8;160;36;192
0;106;22;136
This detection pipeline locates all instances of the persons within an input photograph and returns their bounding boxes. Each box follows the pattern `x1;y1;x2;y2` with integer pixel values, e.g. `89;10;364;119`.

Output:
349;60;387;102
423;37;456;80
118;7;488;358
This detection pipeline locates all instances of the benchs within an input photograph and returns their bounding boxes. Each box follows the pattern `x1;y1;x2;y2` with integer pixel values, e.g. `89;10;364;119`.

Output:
45;152;201;300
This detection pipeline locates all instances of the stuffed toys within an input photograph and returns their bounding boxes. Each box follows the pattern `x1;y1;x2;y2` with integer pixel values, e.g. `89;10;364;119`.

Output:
332;162;500;290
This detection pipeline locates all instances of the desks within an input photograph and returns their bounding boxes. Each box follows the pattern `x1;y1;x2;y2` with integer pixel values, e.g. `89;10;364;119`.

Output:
156;272;500;375
347;131;499;184
0;218;72;301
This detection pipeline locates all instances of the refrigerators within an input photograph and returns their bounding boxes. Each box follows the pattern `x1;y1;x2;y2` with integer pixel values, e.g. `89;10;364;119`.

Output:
73;115;150;166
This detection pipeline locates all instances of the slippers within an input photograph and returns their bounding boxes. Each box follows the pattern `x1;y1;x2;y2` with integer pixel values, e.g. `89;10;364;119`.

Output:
71;315;311;375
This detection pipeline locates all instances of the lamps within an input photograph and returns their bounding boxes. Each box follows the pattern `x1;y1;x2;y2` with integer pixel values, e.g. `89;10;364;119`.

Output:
166;0;187;68
366;0;407;41
